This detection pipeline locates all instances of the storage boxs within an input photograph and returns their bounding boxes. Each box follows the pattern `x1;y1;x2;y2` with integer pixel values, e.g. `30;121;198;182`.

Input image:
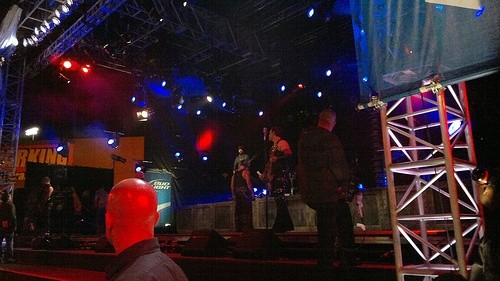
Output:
173;180;481;237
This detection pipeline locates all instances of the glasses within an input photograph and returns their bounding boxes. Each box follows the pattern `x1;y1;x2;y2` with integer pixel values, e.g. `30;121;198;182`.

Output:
41;180;47;184
238;148;246;151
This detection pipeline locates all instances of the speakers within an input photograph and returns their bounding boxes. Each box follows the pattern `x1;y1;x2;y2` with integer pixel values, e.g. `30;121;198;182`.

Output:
233;234;281;260
39;235;74;250
184;230;229;257
96;238;114;252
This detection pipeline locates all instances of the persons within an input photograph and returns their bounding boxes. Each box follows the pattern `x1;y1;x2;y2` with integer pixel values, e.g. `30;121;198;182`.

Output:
1;189;19;264
37;174;109;233
232;144;252;171
296;107;360;279
105;177;190;280
231;159;256;232
261;125;295;230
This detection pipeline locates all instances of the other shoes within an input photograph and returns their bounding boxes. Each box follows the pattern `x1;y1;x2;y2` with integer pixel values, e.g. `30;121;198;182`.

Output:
273;222;295;234
6;257;18;264
337;251;363;268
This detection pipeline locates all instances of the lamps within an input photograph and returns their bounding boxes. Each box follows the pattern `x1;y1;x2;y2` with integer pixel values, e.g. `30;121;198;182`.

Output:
21;0;81;48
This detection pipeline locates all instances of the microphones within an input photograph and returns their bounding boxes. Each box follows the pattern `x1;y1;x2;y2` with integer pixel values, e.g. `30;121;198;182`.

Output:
264;126;268;141
